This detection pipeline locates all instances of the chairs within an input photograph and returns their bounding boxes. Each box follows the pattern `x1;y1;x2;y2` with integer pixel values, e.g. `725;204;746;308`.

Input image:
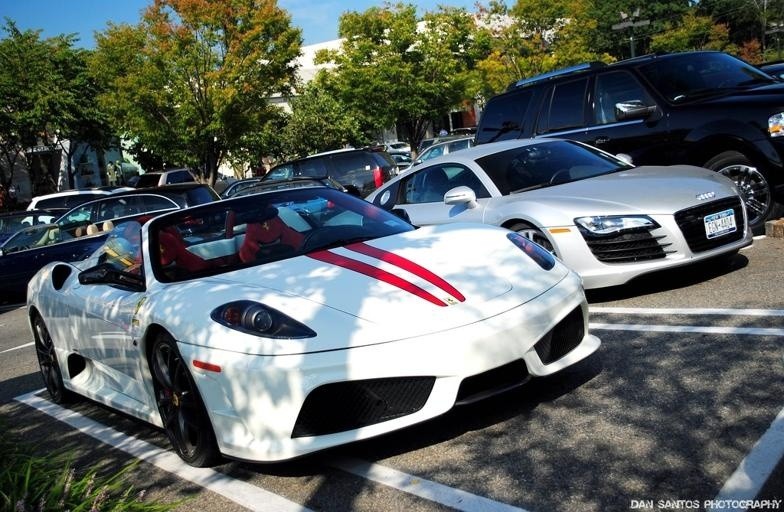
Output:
46;231;58;244
133;232;208;286
422;167;453;201
87;220;114;235
239;213;306;264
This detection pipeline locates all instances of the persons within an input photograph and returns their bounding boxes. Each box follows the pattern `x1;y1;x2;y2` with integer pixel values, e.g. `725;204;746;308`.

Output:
105;161;117;187
113;160;123;187
